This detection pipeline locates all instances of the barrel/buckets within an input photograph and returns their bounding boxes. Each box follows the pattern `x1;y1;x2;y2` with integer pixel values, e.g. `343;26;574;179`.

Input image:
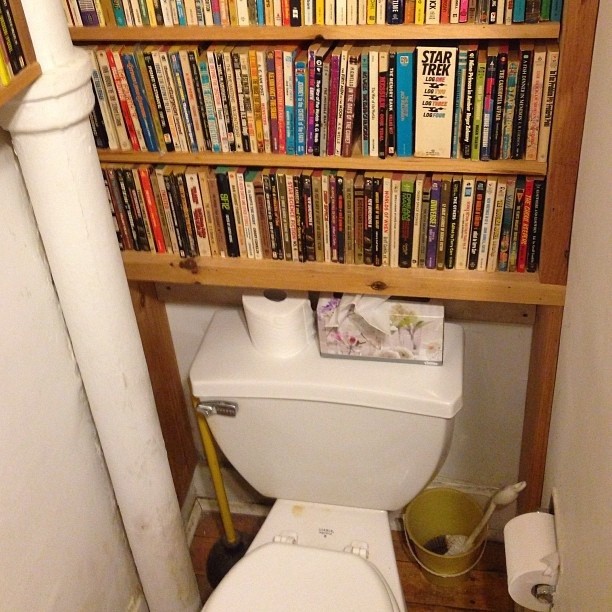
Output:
401;487;492;588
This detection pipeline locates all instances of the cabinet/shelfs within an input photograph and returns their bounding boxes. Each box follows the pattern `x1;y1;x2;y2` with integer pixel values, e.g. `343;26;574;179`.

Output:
61;1;603;307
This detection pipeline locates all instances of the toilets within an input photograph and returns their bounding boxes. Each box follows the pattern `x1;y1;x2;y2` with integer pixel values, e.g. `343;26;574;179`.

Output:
190;311;465;611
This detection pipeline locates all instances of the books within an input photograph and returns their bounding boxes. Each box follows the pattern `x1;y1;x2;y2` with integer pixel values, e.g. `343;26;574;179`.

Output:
83;45;561;163
101;164;547;273
0;0;28;86
62;1;562;27
